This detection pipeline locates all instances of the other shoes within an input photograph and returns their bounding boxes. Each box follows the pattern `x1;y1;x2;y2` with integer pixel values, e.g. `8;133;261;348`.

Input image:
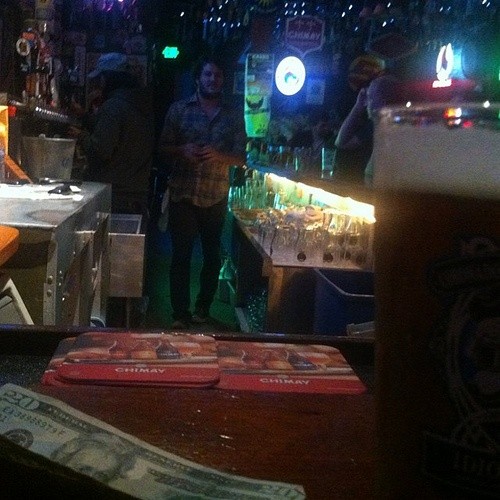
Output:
165;320;188;331
191;310;210;323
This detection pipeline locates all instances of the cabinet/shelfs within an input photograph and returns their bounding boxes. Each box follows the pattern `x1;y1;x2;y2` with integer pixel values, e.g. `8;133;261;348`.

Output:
230;215;319;335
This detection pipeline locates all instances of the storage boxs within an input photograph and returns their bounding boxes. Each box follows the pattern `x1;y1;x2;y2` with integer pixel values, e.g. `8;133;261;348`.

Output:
313;268;377;334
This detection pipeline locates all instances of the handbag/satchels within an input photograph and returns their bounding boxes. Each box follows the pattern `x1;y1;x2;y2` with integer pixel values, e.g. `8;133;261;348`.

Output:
145;167;175;233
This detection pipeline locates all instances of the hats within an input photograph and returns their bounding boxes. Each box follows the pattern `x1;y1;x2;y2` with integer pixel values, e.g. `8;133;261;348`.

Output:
86;53;142;80
346;55;386;92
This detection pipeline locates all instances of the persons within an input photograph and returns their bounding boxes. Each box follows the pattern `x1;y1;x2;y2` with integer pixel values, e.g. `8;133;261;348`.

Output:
158;57;248;328
68;53;155;323
270;55;402;183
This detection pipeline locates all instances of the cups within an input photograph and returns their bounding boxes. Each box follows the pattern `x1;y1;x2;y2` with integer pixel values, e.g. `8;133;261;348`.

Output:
226;142;338;213
366;98;499;500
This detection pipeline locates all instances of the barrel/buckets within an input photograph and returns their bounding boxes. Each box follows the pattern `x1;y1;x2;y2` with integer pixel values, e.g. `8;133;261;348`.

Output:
21;134;77;183
